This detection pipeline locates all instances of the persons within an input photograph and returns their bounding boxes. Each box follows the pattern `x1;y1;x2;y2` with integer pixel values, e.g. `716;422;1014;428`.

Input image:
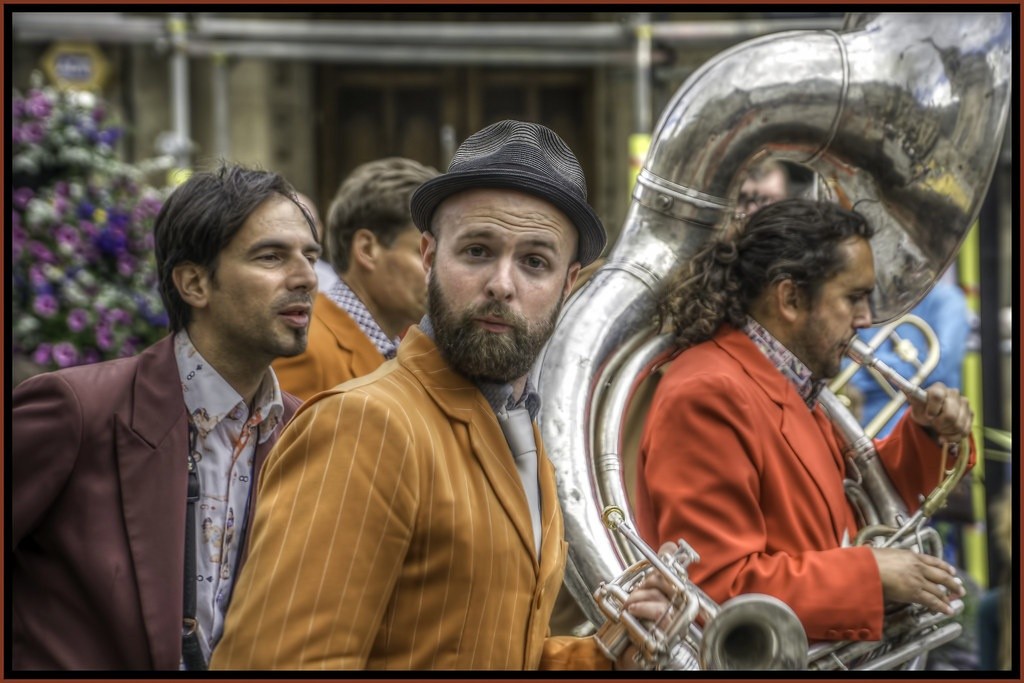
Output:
272;159;444;404
639;194;975;675
840;283;967;556
732;162;786;228
8;157;321;671
207;121;686;671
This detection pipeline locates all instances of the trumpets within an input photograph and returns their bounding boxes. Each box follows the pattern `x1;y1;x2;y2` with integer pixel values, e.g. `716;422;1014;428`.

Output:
579;507;808;672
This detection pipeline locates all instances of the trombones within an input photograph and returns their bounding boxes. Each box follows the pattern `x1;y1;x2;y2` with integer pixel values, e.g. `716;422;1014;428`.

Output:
826;310;943;443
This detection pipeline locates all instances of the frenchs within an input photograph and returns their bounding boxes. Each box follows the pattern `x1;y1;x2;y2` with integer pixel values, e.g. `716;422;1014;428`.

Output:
539;12;1013;673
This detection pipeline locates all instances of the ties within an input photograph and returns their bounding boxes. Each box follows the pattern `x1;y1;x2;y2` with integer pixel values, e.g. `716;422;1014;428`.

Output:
497;406;541;564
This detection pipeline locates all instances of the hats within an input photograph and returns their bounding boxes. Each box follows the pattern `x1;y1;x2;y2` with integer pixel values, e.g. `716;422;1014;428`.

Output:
410;120;607;270
